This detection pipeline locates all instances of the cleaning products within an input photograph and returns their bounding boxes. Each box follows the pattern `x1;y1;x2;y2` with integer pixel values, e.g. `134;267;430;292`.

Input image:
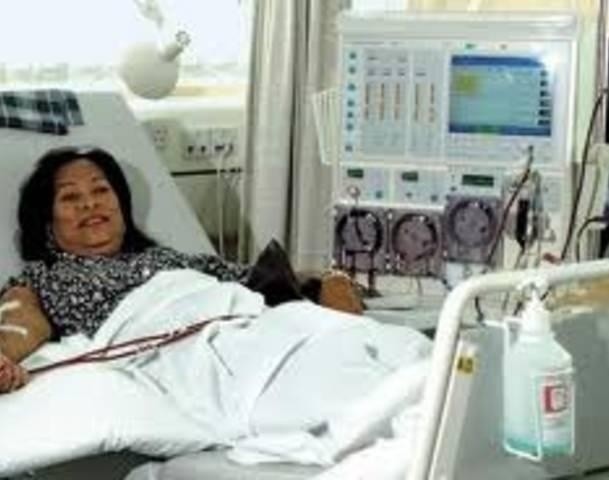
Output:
501;279;577;459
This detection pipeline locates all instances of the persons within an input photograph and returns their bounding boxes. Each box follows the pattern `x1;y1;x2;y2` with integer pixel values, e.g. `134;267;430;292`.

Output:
0;145;364;394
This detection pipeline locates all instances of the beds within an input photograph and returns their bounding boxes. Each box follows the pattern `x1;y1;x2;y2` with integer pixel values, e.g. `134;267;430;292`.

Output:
0;87;608;480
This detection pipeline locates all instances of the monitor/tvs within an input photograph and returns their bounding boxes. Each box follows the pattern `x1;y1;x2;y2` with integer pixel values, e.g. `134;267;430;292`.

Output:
449;54;551;136
